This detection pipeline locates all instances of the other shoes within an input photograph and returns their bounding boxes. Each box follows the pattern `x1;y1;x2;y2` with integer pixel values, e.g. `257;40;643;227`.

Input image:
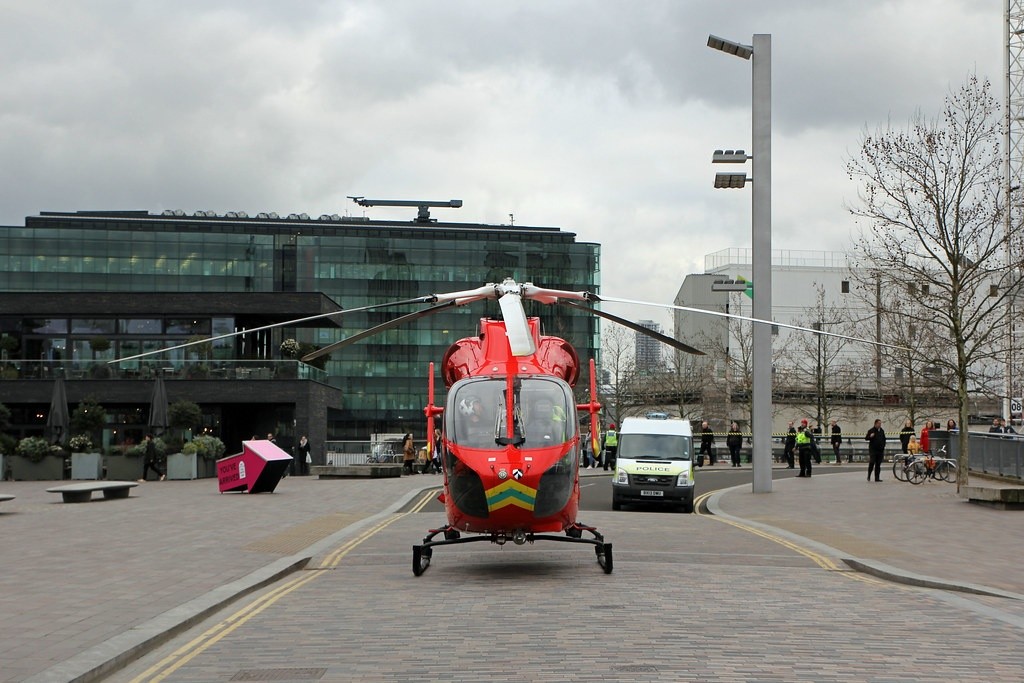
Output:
732;464;736;467
806;475;810;477
796;475;805;477
595;461;599;468
867;476;870;481
737;465;741;467
160;475;165;481
785;466;792;469
875;479;882;482
138;479;145;482
586;465;592;469
707;463;713;466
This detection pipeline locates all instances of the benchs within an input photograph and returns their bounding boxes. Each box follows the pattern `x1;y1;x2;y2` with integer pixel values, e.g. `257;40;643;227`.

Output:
311;463;427;479
960;483;1024;513
46;481;139;503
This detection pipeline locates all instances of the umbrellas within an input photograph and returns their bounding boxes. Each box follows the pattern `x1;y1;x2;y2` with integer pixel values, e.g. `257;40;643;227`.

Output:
47;377;69;445
146;377;173;435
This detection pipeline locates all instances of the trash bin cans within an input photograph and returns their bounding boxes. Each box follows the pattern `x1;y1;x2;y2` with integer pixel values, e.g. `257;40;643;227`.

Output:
928;431;950;471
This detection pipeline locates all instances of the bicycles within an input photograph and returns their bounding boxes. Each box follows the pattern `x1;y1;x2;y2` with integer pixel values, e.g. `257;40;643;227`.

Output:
365;450;397;463
892;444;957;485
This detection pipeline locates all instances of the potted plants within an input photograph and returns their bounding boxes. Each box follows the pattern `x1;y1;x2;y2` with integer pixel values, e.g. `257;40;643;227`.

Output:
0;436;226;482
1;368;19;380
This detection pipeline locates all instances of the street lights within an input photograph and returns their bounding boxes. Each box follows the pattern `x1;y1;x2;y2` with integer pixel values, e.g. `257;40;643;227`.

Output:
706;31;774;495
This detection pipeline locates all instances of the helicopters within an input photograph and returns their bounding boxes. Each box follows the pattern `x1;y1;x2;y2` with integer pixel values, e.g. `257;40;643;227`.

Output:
106;275;914;579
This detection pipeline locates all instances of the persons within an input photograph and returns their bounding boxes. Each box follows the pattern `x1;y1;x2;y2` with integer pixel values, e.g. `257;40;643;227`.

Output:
401;429;443;475
988;418;1018;440
251;435;258;440
865;419;887;483
726;423;743;467
783;419;822;478
695;422;716;467
907;435;920;455
137;433;166;482
830;420;842;464
601;423;619;471
899;420;916;466
298;436;311;476
946;419;958;433
584;424;600;470
920;421;936;470
267;434;277;445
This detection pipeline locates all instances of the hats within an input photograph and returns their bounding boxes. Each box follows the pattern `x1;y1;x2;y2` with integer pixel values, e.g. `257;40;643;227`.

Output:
610;423;615;428
801;420;807;424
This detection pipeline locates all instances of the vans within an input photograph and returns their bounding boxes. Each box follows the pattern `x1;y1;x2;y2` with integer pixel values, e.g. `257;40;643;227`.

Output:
605;412;704;514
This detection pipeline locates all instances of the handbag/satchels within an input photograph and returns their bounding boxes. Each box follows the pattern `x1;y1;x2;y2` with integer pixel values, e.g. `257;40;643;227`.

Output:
305;452;312;463
